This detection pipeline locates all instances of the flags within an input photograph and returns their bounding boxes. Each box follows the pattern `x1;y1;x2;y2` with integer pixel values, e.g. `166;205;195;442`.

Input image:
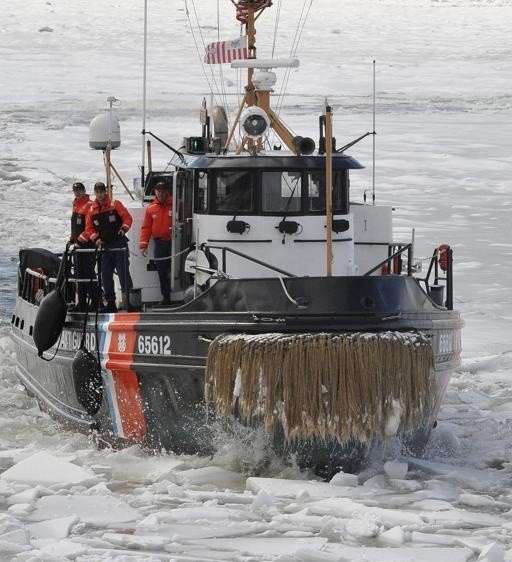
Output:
204;35;248;64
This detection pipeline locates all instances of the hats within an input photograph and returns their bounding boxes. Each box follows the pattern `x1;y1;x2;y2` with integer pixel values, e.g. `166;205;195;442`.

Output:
72;183;85;191
94;182;106;191
155;182;168;190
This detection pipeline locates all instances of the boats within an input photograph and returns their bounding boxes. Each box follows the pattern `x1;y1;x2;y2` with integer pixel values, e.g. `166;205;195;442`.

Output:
11;2;466;481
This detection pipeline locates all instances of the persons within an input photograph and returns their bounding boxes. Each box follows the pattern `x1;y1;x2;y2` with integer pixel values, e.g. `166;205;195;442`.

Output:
84;182;140;313
68;182;106;312
139;182;184;306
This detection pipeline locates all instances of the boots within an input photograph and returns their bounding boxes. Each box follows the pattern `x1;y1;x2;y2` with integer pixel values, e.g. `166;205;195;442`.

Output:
158;292;172;305
87;298;99;312
97;296;118;313
67;298;86;312
